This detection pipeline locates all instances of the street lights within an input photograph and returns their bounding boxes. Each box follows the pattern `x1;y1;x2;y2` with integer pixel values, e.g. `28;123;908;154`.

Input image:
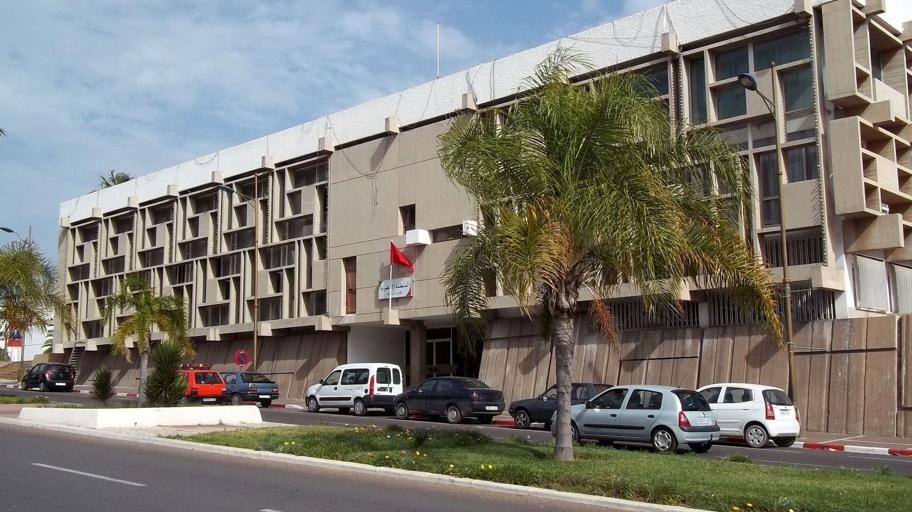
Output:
215;170;258;374
737;61;795;410
0;223;32;388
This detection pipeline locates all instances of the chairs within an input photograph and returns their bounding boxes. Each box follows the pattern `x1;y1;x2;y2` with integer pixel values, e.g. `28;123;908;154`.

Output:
628;393;643;409
724;392;733;403
741;395;749;402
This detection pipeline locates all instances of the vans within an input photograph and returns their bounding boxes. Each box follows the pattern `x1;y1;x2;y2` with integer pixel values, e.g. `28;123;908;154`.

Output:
306;363;404;418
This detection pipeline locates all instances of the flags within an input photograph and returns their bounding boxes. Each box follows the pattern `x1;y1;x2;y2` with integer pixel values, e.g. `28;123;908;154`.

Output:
389;241;413;307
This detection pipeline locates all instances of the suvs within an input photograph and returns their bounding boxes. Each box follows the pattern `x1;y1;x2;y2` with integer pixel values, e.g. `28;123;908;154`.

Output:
173;364;228;405
21;362;76;393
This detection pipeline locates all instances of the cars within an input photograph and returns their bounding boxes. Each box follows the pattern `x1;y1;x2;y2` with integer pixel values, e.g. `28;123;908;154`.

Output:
694;383;802;450
392;375;505;425
219;371;279;408
508;382;617;429
549;384;720;453
